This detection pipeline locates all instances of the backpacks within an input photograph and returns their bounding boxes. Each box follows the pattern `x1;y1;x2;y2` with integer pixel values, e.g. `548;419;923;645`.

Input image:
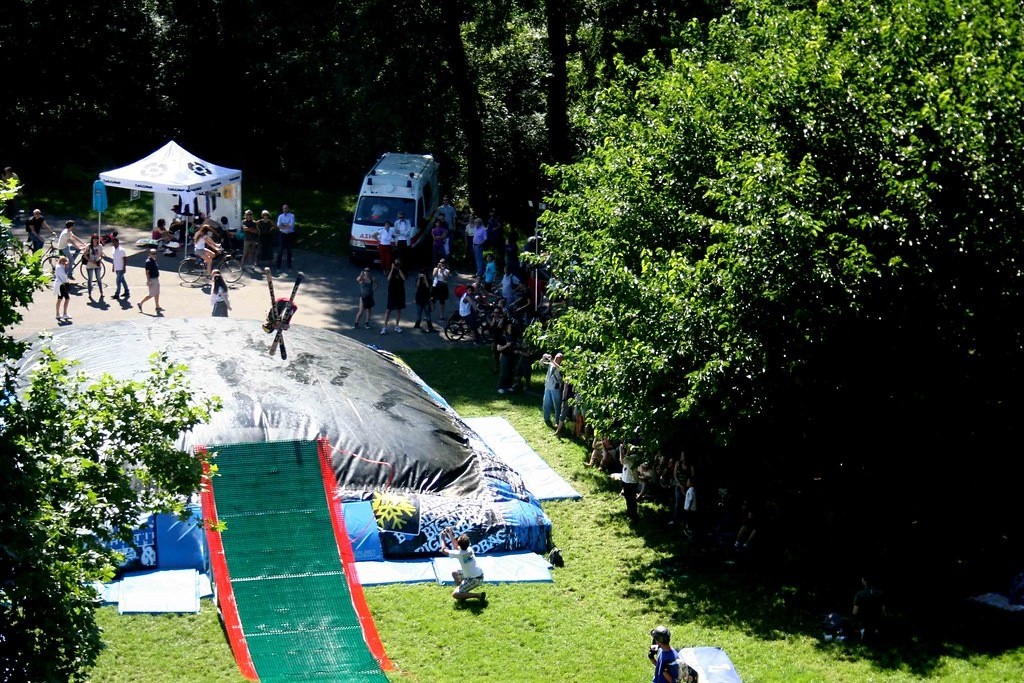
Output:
668;659;698;683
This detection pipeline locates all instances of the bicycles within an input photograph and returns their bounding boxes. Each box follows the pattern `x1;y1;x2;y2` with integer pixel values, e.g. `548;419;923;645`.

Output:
41;247;106;282
445;296;502;343
14;236;61;267
179;247;243;284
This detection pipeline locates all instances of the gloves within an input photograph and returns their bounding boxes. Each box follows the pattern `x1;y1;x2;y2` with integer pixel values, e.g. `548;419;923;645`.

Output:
662;660;671;672
649;649;657;659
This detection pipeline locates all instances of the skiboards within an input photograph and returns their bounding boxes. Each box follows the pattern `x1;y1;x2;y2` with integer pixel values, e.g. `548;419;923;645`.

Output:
264;265;305;360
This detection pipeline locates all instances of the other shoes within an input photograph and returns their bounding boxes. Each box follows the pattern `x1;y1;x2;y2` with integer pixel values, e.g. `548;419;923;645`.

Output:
352;325;358;330
480;592;485;606
365;324;371;329
121;292;130;297
137;303;142;312
63;314;72;319
156;307;165;311
56;315;62;319
110;295;119;299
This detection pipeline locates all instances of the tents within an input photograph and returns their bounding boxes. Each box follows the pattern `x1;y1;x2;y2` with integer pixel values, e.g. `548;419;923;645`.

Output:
99;140;242;258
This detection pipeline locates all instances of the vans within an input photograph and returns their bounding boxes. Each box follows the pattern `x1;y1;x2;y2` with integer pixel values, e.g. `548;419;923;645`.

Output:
349;152;441;269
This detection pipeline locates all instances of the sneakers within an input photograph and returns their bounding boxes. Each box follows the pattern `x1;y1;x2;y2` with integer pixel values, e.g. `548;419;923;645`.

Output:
395;327;403;333
381;328;388;335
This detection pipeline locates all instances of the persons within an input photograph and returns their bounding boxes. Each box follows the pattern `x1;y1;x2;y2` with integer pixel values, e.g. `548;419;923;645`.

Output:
242;205;295;272
111;238;130;297
152;214;237;279
540;352;761;548
54;257;72;319
649;625;682;683
84;234;104;298
211;271;232;317
137;250;164;312
3;166;23;222
352;195;529;393
442;528;486;603
58;221;87;280
29;209;57;253
102;231;118;244
839;575;877;644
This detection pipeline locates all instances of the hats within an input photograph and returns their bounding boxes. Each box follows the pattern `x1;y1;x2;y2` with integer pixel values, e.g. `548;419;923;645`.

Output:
398;212;406;217
654;626;670;642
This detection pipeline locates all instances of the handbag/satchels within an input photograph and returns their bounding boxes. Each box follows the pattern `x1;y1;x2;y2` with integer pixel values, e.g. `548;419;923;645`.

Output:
362;294;375;308
63;283;72;293
82;245;90;265
436;282;448;299
